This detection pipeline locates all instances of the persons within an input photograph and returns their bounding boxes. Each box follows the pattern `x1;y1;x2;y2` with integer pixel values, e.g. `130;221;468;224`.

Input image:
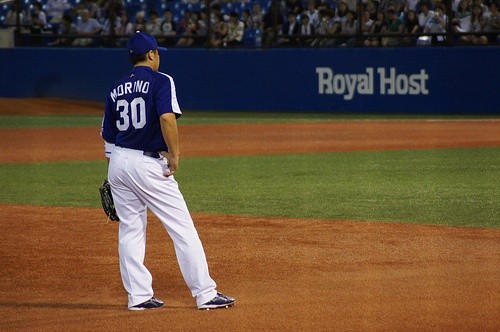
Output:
1;0;500;47
100;33;237;311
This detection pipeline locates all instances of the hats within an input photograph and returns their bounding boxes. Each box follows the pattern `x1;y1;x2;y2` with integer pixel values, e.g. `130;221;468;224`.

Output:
126;31;167;56
135;12;145;19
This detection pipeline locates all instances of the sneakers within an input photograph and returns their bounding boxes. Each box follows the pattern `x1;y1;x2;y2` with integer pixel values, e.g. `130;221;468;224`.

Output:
127;296;164;312
195;291;236;310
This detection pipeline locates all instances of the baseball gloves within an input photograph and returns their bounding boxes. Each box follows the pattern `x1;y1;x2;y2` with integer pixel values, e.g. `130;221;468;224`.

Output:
98;177;120;222
